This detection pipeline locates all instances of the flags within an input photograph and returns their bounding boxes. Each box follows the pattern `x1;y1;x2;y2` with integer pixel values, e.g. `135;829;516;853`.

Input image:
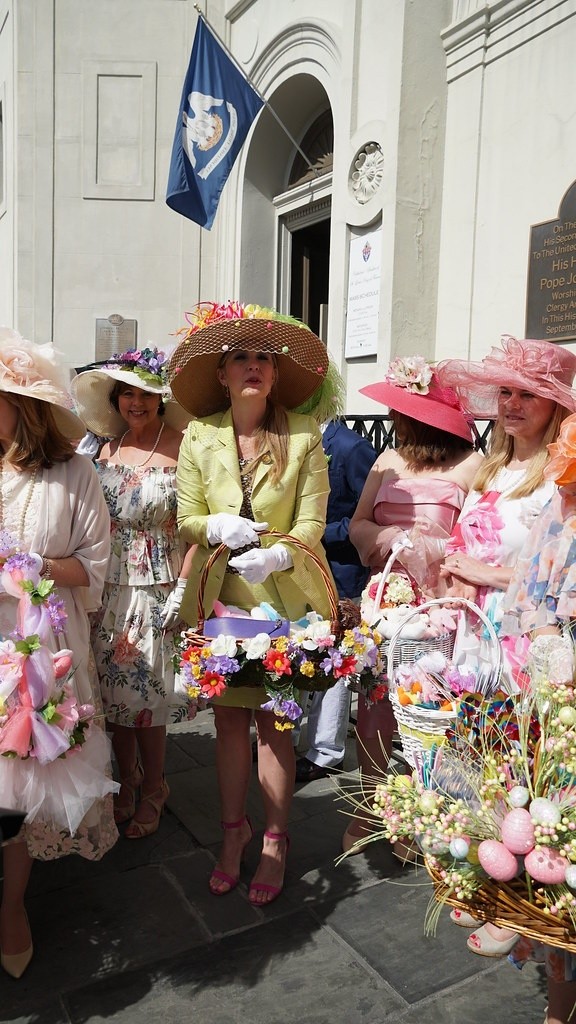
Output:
166;15;264;235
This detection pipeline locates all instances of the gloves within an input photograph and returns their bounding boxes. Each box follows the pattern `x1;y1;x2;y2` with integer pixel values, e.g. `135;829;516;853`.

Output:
206;512;268;549
161;578;188;630
229;542;293;586
393;530;453;572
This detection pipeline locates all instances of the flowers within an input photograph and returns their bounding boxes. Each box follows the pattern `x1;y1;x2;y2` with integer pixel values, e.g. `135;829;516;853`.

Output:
0;300;576;938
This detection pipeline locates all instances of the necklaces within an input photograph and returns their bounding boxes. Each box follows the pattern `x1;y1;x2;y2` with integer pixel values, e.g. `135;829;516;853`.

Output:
0;468;36;552
117;422;164;466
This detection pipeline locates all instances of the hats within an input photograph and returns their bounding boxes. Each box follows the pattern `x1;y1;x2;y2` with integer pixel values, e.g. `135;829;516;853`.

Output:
0;354;86;439
169;319;329;420
359;366;476;443
432;336;576;421
69;354;195;437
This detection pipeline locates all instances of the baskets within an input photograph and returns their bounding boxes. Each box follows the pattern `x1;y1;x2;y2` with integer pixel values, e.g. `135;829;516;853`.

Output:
384;596;502;769
183;530;341;690
425;734;575;951
355;544;456;669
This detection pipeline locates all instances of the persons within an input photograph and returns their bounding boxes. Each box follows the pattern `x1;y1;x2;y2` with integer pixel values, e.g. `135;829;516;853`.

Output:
166;302;340;906
437;333;576;1024
0;327;111;978
251;413;378;782
342;355;485;862
70;347;212;837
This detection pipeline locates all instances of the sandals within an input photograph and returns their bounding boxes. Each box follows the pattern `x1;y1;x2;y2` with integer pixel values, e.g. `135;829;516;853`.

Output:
466;922;523;957
449;907;485;928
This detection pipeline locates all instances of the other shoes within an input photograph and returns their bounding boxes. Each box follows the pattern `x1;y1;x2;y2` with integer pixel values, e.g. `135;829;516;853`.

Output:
0;903;34;978
292;757;344;783
341;820;373;856
394;840;420;863
250;739;258;761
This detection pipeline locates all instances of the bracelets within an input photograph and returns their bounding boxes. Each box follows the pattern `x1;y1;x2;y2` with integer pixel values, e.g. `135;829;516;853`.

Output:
41;557;52;580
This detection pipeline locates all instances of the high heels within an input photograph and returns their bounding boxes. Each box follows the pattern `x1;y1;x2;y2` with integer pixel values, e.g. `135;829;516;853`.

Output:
124;772;171;838
208;816;254;896
110;757;147;824
248;828;292;905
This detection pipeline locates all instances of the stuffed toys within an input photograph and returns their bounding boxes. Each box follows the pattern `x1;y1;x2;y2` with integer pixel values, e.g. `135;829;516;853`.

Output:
360;572;457;640
